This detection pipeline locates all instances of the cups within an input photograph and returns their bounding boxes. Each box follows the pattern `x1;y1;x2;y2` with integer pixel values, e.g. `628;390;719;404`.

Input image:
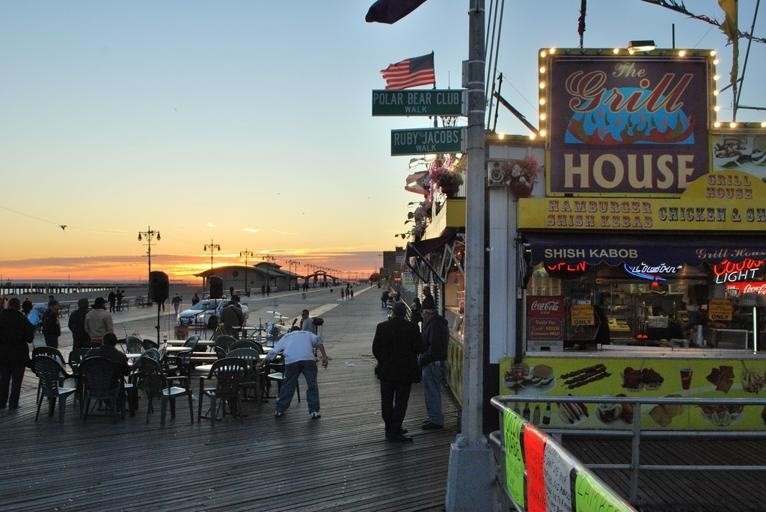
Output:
680;368;694;391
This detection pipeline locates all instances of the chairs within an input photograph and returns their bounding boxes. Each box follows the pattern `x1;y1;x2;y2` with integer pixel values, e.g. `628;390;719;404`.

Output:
27;333;293;428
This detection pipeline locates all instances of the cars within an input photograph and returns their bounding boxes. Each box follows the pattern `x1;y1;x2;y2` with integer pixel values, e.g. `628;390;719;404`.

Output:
176;299;249;328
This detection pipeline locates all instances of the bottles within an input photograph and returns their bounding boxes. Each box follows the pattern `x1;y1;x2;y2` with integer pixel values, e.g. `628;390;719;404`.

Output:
163;335;168;347
514;400;552;426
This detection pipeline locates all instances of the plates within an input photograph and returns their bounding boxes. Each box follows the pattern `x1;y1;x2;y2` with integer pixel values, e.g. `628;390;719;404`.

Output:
505;366;557;394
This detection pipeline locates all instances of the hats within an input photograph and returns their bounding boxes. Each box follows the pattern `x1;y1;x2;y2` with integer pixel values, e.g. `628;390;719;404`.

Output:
95;297;107;305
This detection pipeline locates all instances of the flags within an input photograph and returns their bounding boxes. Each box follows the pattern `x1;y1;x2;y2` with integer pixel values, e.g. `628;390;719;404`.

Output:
380;53;434;90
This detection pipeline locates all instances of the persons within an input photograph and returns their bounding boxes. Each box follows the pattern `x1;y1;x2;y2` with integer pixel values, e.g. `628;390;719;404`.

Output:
0;297;9;310
341;282;353;300
301;309;314;333
247;285;251;297
260;326;328;418
23;289;126;423
381;289;401;308
171;293;182;315
1;299;33;408
372;302;419;443
419;308;449;430
192;293;199;305
411;286;434;325
205;286;243;340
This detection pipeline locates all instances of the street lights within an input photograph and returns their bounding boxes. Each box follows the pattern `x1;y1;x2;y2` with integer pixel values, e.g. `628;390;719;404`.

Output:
139;226;371;301
395;158;432;241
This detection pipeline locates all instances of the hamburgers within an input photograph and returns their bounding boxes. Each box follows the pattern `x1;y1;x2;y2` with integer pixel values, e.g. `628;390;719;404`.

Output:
527;364;559;393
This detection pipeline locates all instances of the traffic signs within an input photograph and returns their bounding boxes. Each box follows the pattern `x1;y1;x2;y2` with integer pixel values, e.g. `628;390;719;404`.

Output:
392;128;461;155
372;90;462;114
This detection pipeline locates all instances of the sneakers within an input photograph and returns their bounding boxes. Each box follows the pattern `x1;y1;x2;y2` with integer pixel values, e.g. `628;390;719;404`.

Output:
385;427;412;443
0;403;21;409
422;419;443;429
274;410;282;416
310;412;321;418
